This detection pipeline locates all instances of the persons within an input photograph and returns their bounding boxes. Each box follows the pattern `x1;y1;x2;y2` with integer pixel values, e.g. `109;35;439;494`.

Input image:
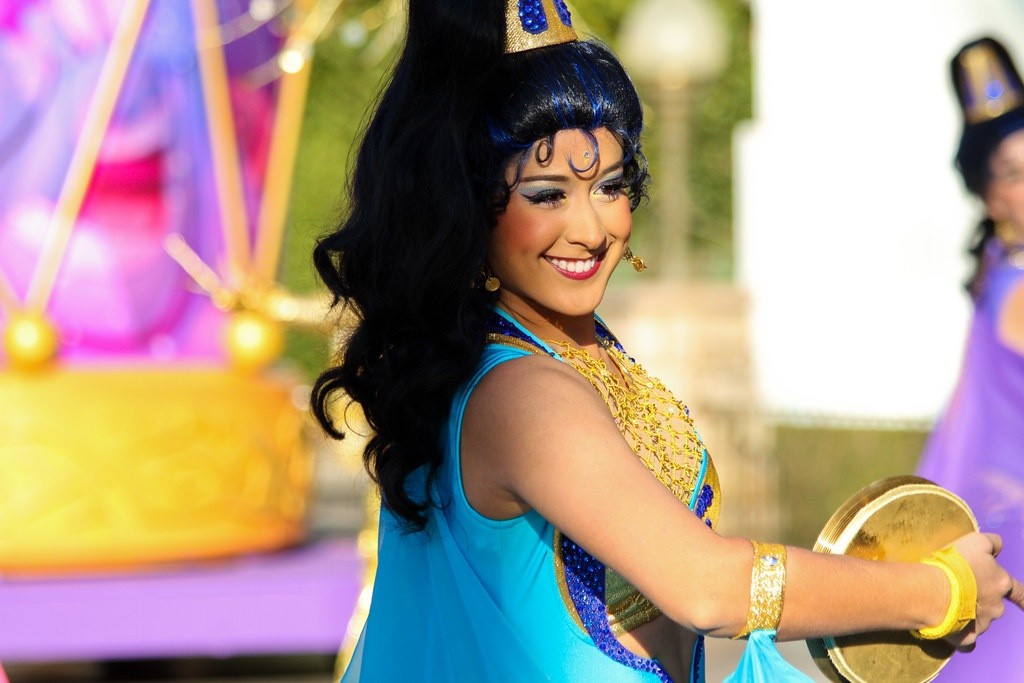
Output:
309;1;1024;683
916;38;1024;683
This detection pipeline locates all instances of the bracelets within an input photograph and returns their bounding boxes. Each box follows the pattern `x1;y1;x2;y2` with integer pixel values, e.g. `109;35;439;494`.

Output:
730;540;786;640
911;547;977;640
